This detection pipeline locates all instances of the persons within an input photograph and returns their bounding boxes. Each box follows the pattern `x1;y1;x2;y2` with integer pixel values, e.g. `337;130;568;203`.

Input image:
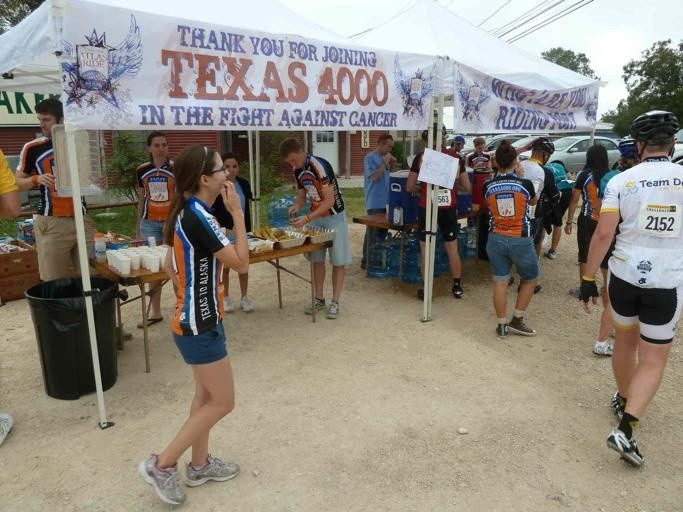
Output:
579;109;683;467
279;137;351;319
18;98;81;285
211;152;254;313
135;143;241;505
134;133;177;329
0;123;23;450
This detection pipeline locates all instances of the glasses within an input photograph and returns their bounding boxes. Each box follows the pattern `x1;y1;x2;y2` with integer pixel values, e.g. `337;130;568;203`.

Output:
204;166;235;174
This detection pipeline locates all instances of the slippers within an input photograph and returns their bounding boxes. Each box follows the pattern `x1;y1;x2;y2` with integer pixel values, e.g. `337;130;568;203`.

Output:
137;313;166;327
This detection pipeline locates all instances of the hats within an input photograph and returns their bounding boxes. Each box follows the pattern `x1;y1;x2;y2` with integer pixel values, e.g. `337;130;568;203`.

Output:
453;134;465;143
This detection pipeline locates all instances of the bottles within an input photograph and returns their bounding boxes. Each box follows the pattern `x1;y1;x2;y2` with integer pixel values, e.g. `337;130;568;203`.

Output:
21;219;34;246
94;233;106;259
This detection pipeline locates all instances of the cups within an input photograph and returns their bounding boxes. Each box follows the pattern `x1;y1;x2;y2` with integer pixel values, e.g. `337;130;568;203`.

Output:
107;243;167;274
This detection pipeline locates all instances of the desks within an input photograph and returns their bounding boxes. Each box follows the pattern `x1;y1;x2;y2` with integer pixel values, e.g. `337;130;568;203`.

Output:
349;206;486;279
86;233;333;374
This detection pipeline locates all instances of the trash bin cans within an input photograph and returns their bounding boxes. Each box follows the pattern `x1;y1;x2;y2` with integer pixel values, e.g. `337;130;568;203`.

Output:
24;273;119;400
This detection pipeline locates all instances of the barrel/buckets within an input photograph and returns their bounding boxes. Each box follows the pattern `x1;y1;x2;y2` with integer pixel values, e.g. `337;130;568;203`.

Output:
454;166;474;217
471;166;495;210
367;219;477;285
267;193;311;228
388;169;420;225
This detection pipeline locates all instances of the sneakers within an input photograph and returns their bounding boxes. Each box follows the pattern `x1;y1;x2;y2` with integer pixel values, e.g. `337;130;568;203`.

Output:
545;249;555;259
494;317;537;339
327;300;341;320
568;286;584;300
240;295;258;313
222;295;235;313
304;297;326;318
590;338;614;358
610;390;629;422
137;453;187;507
183;452;241;490
449;285;466;299
504;275;544;296
604;428;646;468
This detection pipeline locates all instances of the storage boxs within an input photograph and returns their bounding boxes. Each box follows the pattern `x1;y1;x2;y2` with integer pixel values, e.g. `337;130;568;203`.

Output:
0;272;42;305
0;237;38;276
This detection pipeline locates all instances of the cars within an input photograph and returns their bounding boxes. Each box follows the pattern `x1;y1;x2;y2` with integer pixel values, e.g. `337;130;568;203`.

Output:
670;129;683;165
613;136;638;161
445;134;564;170
516;135;621;175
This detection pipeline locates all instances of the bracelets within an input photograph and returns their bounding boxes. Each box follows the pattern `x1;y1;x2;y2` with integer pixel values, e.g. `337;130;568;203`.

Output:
306;214;311;223
32;175;38;185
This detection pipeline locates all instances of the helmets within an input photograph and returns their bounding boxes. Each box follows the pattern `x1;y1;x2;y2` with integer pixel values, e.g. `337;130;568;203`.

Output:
628;108;682;148
532;136;558;156
617;138;639;159
